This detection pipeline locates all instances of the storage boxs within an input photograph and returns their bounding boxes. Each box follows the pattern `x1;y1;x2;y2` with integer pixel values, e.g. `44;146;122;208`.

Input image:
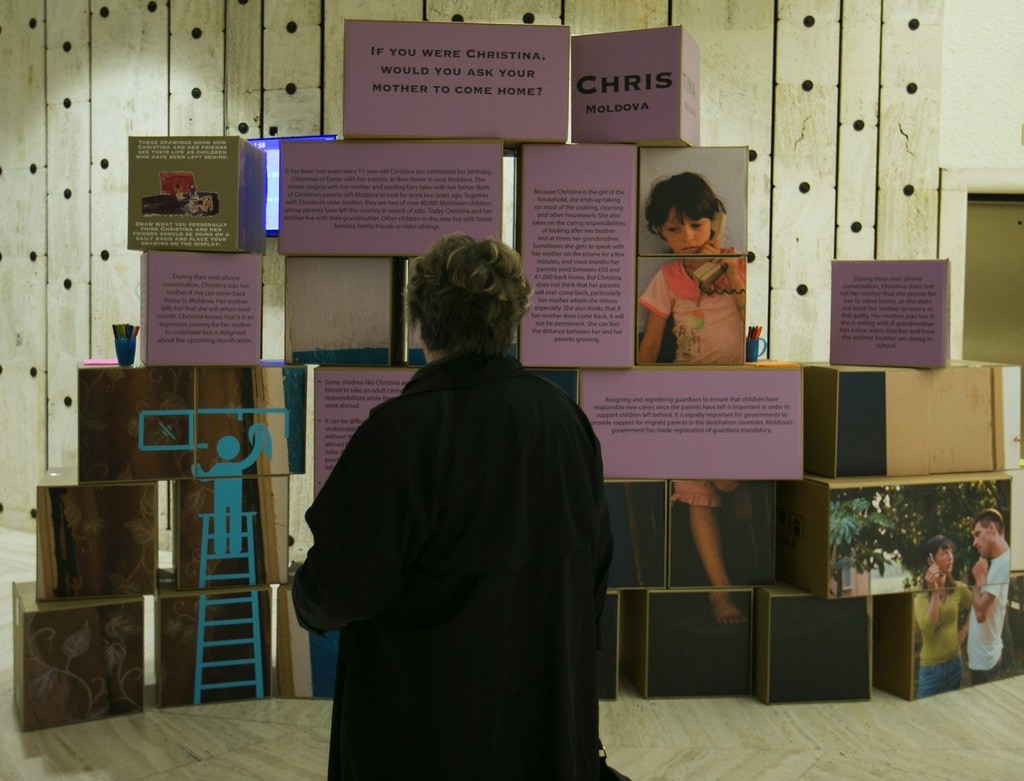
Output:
153;568;271;708
75;362;197;484
36;467;158;601
127;135;268;255
12;580;144;734
138;251;264;368
196;366;308;479
280;15;1024;706
170;475;288;591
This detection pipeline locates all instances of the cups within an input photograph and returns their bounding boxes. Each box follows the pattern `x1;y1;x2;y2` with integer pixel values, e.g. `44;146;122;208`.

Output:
115;338;136;365
746;338;766;362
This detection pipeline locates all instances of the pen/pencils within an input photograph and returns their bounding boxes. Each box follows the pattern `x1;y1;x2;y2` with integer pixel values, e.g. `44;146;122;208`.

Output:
111;324;140;339
747;325;763;339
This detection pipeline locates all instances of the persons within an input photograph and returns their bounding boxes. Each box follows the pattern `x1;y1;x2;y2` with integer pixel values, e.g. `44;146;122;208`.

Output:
292;236;631;781
913;509;1011;699
637;170;750;620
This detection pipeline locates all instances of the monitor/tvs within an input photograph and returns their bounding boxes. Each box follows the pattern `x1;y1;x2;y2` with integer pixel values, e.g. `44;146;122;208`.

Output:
245;134;339;238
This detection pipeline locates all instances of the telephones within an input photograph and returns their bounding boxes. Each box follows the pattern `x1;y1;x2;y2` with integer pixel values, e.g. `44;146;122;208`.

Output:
691;211;725;287
